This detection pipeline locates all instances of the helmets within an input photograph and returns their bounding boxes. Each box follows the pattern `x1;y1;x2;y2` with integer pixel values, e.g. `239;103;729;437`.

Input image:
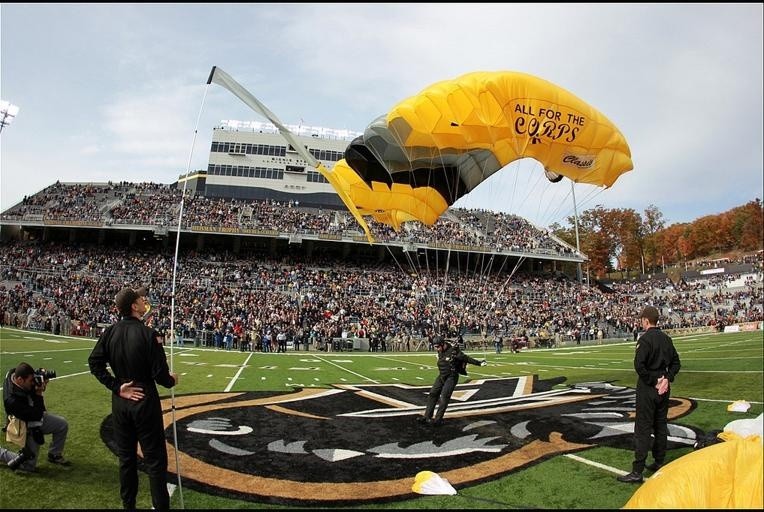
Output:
431;337;447;346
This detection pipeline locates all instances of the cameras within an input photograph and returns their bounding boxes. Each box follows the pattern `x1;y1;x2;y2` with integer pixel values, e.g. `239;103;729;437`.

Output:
33;367;57;382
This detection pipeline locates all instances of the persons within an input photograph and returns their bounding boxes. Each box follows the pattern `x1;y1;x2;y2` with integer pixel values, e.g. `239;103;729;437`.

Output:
510;338;521;354
415;333;488;423
617;306;681;481
0;362;73;471
0;253;763;351
0;180;577;249
88;287;178;510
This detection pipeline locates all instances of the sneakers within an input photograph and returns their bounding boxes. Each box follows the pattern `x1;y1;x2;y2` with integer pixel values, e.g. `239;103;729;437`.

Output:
50;453;69;465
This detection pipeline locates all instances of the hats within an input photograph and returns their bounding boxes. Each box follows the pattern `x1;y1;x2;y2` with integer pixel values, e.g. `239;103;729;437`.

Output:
115;283;147;310
635;306;659;318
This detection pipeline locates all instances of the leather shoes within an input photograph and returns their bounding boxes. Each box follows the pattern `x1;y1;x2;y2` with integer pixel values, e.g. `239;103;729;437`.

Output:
617;474;644;482
415;415;428;423
428;418;441;425
647;462;663;471
